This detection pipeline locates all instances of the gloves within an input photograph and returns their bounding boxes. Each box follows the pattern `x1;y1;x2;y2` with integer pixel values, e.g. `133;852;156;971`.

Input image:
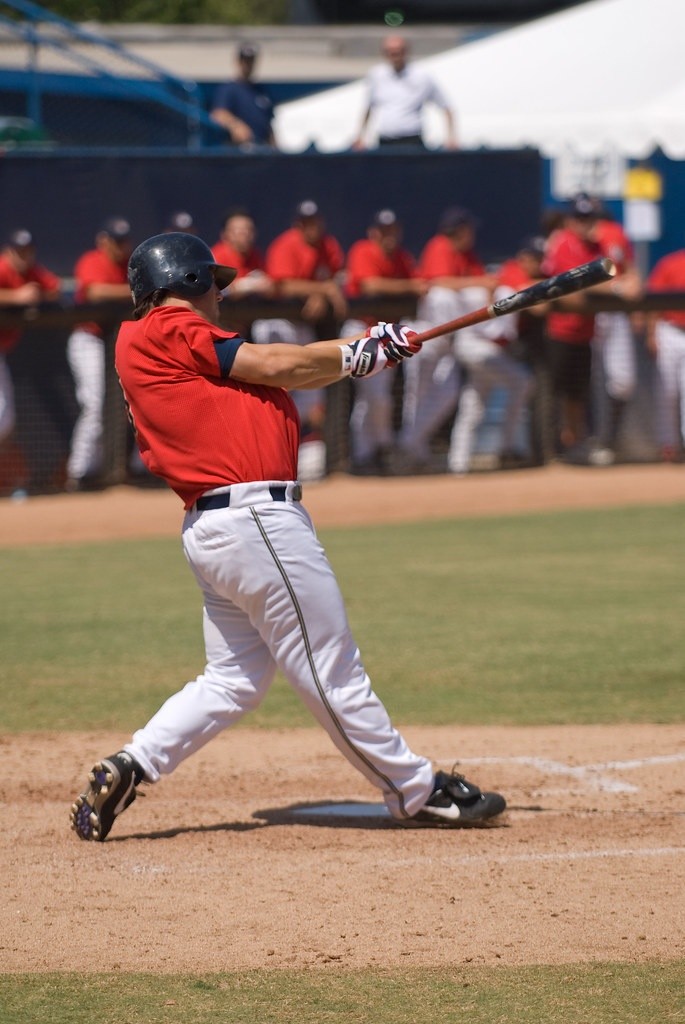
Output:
365;322;422;363
339;337;388;379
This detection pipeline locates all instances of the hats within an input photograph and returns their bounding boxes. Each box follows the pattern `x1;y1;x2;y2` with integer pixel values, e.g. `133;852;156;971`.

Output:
235;43;258;58
168;211;197;230
9;228;33;249
297;200;321;219
567;193;595;216
382;37;405;51
371;209;398;229
529;234;548;258
439;207;478;228
104;216;130;240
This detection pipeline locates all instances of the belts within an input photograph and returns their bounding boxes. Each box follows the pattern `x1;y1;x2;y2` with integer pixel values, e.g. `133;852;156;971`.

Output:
196;485;302;511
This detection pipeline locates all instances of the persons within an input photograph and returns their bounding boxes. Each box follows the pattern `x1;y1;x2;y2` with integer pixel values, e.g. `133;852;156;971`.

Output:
249;203;347;423
69;232;508;840
0;228;61;438
644;247;685;463
353;37;458;152
210;212;275;302
208;46;277;146
338;210;428;477
402;192;644;474
65;216;134;492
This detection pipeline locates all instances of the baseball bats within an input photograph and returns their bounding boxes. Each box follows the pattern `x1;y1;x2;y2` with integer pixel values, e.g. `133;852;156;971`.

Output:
406;256;616;345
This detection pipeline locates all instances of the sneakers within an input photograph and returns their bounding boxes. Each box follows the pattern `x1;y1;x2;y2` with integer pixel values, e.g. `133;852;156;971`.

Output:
68;751;149;842
392;762;506;827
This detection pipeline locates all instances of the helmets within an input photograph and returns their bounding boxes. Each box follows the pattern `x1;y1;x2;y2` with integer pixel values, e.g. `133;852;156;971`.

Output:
128;232;237;308
